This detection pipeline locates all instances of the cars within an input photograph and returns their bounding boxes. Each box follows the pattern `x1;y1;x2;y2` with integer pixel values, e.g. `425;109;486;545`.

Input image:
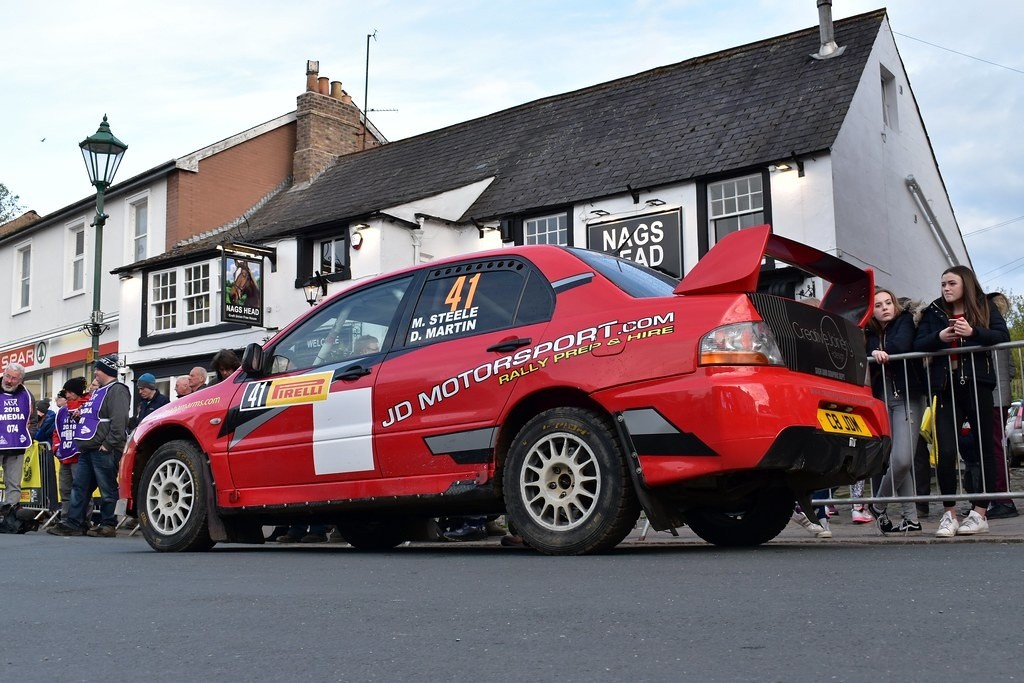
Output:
115;225;892;553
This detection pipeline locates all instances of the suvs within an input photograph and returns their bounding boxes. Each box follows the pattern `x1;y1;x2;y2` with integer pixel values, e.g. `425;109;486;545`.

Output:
1005;399;1024;467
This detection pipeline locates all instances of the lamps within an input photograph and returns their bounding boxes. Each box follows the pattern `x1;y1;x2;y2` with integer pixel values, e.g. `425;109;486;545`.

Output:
303;271;327;307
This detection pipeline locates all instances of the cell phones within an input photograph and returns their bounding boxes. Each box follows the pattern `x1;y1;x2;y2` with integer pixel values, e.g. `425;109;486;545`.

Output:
948;319;959;333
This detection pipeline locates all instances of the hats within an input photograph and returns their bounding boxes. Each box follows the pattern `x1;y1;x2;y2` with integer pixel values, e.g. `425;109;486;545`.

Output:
94;352;119;377
63;376;87;396
57;389;67;399
136;373;158;390
35;397;51;414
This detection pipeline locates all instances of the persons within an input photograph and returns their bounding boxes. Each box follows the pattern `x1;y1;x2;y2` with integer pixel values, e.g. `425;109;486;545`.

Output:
912;264;1011;540
47;351;131;538
862;288;928;539
987;292;1020;522
208;347;242;387
70;376;101;424
174;374;194;398
0;363;38;505
46;376;92;536
126;372;172;529
353;334;379;354
34;397;57;513
896;295;936;520
277;349;302;374
52;390;67;411
789;477;874;539
188;366;208;394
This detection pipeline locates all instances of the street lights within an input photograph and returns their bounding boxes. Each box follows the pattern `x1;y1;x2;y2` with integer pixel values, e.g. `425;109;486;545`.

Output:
79;112;130;379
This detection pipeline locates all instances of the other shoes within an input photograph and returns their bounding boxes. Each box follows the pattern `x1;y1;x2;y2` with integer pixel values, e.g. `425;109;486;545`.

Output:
916;508;930;518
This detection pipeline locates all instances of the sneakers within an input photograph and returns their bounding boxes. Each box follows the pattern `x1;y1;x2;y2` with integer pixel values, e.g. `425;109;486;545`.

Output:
850;507;874;523
827;504;839;516
884;518;922;537
935;510;990;537
47;523;83;536
87;524;116;537
790;509;832;538
985;502;1019;519
868;503;893;535
